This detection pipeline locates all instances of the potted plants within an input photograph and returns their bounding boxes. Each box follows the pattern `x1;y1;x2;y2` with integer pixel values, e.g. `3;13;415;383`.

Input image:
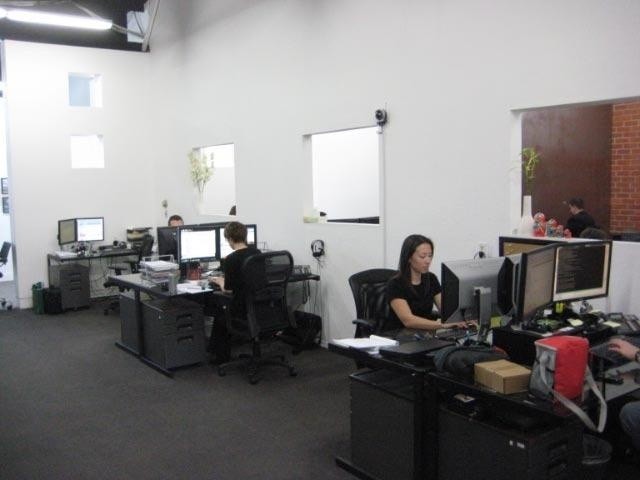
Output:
518;145;541;234
187;151;213;212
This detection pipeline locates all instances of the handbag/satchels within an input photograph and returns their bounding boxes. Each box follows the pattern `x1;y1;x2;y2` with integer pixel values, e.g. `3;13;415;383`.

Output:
635;350;639;361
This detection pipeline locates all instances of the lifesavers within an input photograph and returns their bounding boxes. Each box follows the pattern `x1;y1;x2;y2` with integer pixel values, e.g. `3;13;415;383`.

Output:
493;314;598;367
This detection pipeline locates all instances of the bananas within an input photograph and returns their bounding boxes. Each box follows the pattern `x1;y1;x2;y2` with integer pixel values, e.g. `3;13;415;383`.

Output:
589;336;640;364
437;326;478;341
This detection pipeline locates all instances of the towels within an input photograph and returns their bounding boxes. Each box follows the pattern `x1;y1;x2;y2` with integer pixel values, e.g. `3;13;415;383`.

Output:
581;434;613;480
43;288;60;314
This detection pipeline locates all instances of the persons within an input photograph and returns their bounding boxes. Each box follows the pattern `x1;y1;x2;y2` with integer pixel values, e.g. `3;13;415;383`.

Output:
564;198;593;237
375;234;465;332
167;215;184;226
608;336;640;455
577;227;606;239
203;221;264;364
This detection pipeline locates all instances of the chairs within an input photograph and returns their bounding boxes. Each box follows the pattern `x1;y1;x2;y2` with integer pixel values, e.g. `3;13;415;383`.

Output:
104;234;153;313
348;268;404;338
214;251;297;383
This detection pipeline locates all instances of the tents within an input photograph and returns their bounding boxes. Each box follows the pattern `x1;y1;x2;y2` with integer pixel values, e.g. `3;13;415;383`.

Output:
311;239;324;257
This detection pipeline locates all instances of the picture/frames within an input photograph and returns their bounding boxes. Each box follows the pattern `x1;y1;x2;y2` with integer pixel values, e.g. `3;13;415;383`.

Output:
110;271;322;375
48;245;156;312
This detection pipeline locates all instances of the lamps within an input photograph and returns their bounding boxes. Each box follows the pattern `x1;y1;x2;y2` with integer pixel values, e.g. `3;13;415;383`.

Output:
214;251;297;383
348;268;404;338
104;234;153;313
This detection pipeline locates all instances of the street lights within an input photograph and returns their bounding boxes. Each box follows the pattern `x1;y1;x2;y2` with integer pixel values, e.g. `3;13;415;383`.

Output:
433;345;508;374
529;336;588;404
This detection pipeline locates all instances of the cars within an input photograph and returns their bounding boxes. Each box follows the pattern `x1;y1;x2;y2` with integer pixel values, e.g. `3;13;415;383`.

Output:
188;259;202;280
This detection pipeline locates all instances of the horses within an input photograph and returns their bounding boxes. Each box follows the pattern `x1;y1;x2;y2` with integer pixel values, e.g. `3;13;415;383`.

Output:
599;372;624;384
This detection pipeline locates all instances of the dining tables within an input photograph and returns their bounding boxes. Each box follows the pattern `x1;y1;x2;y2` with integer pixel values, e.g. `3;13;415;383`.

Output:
518;145;541;234
187;151;213;212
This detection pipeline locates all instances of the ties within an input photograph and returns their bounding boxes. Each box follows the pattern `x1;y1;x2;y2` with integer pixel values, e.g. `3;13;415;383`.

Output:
441;235;506;345
499;235;568;258
157;222;256;278
58;217;104;250
507;243;557;321
552;240;612;303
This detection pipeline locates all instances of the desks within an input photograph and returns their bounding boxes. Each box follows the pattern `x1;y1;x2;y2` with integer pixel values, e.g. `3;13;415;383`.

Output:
328;311;640;480
110;271;322;375
48;245;156;312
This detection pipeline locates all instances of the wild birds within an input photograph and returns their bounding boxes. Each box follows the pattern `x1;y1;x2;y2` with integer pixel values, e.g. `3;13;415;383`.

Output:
379;339;456;367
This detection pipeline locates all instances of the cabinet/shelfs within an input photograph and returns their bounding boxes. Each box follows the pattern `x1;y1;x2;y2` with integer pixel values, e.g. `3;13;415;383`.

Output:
532;213;572;240
